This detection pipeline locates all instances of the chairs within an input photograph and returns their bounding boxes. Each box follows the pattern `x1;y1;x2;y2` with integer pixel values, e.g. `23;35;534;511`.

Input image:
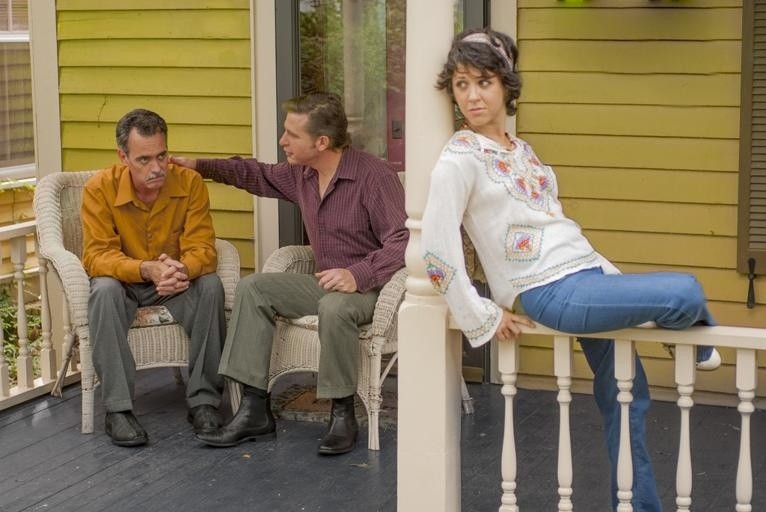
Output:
31;172;247;430
250;172;474;448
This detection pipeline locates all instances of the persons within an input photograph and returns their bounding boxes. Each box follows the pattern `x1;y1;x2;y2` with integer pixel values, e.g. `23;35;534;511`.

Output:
82;110;226;446
168;91;410;454
422;29;721;512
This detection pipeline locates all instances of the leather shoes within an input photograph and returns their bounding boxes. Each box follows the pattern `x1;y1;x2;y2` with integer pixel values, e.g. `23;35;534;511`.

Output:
105;412;148;446
665;345;723;372
188;404;224;432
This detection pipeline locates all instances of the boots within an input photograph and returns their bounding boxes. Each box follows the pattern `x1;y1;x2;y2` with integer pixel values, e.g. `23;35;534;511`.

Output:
196;396;278;447
318;398;358;456
